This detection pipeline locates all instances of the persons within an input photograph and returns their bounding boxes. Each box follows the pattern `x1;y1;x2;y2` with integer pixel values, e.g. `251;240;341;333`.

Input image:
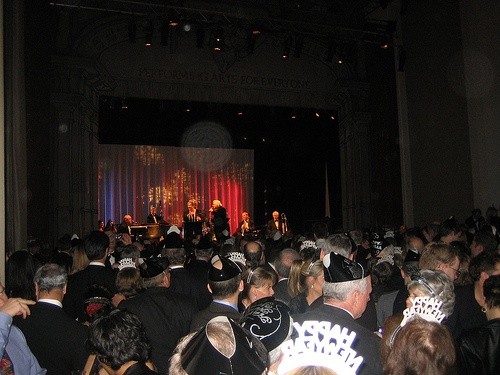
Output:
0;199;500;375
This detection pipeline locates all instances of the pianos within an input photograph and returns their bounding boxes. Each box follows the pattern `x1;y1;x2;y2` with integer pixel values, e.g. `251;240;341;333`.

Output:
130;223;182;244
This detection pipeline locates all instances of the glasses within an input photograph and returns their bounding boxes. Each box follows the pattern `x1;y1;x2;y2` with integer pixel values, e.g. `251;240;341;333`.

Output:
450;267;461;276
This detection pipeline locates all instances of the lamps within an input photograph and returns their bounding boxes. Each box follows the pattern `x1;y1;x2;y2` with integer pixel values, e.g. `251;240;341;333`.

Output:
294;33;304;58
145;17;155;47
246;31;257;45
213;29;223;51
194;24;205;49
328;41;335;62
127;18;137;44
280;35;293;58
159;18;169;45
